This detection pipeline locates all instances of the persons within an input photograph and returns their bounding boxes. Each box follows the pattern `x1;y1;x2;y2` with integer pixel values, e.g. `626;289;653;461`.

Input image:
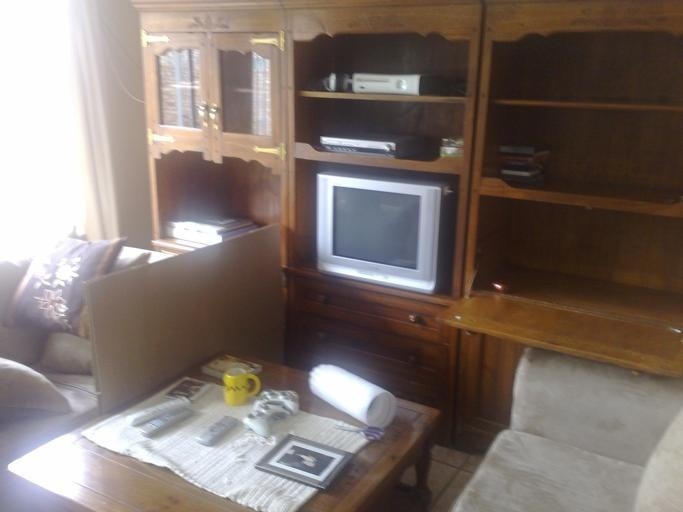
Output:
277;448;316;467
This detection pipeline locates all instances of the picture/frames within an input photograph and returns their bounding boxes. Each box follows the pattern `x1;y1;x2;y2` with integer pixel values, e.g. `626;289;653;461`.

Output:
253;434;353;490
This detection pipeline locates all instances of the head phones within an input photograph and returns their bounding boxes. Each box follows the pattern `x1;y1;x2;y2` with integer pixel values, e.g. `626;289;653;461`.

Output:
321;72;351;92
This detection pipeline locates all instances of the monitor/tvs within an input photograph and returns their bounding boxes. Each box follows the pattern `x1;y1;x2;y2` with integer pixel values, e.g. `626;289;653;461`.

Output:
314;171;456;295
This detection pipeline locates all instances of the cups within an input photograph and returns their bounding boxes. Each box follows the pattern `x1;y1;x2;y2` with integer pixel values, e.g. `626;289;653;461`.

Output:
222;367;261;408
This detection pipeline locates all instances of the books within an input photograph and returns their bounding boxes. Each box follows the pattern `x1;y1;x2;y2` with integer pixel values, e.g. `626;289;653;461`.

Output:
201;353;263;381
163;215;258;245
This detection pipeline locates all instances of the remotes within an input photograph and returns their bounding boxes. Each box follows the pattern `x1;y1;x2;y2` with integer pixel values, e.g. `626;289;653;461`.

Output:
125;396;191;427
196;415;240;447
138;407;192;437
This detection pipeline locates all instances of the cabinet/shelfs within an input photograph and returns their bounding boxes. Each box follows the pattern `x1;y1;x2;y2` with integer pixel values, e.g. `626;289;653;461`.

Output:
137;5;289;267
291;5;681;448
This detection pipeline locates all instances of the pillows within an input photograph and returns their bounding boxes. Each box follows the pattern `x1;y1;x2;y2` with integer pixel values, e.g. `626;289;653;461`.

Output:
3;223;151;376
1;358;75;424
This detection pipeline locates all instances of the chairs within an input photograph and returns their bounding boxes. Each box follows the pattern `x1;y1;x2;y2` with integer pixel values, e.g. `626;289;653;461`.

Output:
449;344;683;512
